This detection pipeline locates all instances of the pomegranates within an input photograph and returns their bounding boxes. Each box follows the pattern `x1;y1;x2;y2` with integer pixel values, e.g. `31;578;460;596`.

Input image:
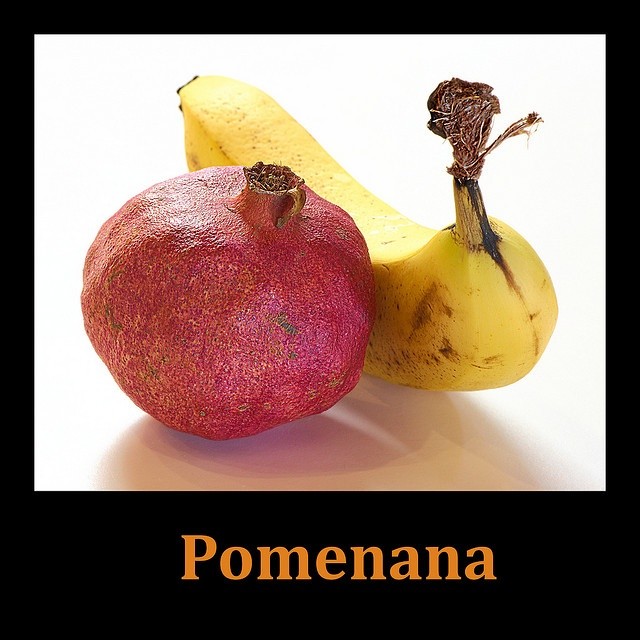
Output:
81;161;376;442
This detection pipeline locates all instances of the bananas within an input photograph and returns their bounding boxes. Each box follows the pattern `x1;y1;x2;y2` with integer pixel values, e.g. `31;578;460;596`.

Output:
178;74;557;390
